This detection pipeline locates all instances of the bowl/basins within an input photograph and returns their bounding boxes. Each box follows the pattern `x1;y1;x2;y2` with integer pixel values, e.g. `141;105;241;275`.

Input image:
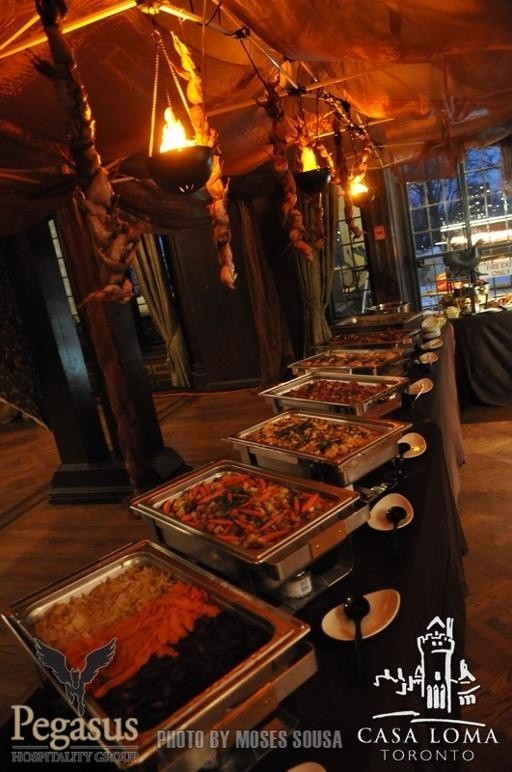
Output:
443;248;481;271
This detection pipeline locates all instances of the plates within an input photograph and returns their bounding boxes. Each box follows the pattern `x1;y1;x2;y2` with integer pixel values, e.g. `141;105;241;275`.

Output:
319;587;399;643
461;292;511;314
405;315;444;400
368;493;415;535
396;434;428;458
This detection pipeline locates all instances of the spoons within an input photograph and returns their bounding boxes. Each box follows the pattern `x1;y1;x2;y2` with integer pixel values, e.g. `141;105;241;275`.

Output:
342;592;370;648
386;504;409;538
396;442;410;472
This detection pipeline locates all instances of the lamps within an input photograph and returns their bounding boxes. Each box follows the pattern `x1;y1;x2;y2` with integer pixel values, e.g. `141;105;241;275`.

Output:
147;28;213;196
292;93;332;194
350;136;369;208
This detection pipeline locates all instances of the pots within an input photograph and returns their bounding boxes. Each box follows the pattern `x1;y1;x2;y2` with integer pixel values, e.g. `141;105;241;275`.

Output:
368;301;409;315
